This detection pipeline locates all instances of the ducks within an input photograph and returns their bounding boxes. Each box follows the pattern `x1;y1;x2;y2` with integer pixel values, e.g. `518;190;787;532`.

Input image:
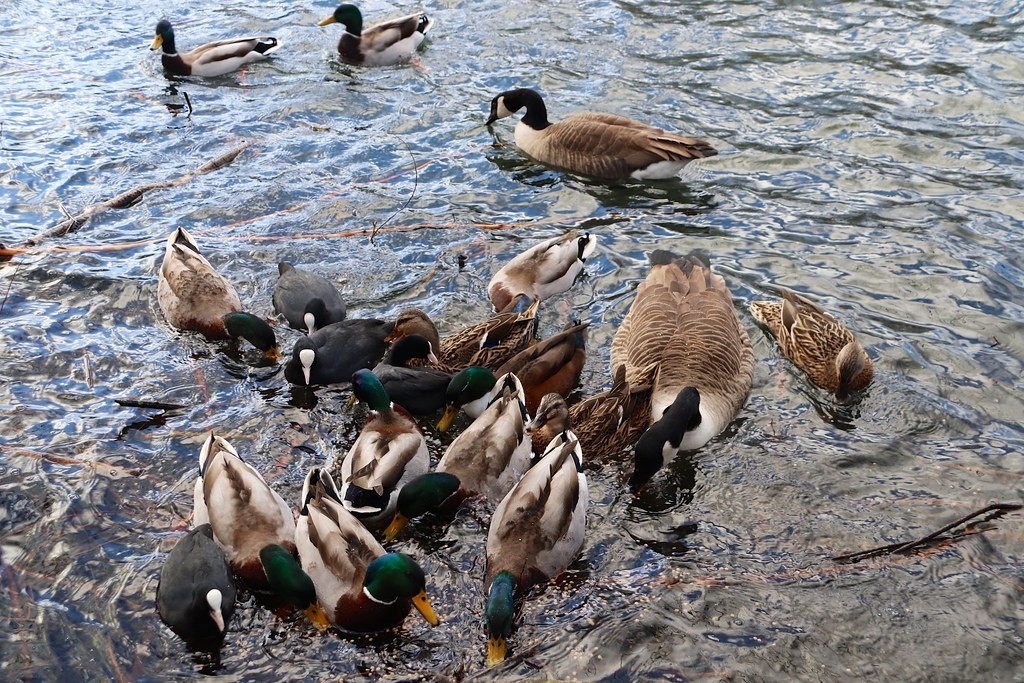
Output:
317;4;435;64
155;225;651;668
150;20;284;77
750;287;874;402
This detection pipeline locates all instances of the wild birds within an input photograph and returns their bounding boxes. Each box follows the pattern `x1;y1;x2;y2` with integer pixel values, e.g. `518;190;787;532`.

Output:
483;89;719;179
613;249;754;493
